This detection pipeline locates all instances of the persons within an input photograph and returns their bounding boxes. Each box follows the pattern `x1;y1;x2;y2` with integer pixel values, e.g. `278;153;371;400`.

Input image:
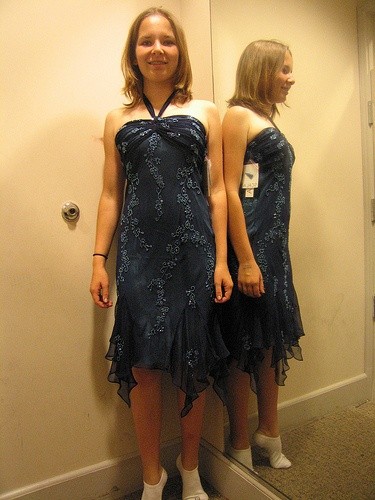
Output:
223;40;306;473
90;7;233;500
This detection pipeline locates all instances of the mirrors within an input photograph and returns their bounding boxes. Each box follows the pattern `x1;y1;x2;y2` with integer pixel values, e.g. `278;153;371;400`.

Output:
210;0;375;499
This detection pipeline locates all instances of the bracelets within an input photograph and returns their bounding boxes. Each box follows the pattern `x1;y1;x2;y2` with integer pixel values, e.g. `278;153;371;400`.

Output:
93;253;106;258
